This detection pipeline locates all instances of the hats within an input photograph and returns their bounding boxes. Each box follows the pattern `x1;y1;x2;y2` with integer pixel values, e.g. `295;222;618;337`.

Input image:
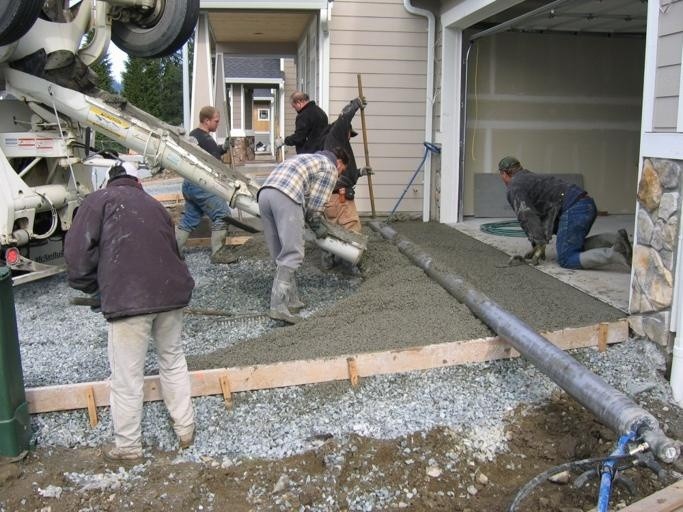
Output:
106;166;139;183
331;119;359;138
497;155;521;172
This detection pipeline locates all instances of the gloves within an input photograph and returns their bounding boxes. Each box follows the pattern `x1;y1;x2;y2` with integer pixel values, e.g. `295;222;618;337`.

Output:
359;166;376;176
525;242;546;266
357;95;368;110
90;289;102;313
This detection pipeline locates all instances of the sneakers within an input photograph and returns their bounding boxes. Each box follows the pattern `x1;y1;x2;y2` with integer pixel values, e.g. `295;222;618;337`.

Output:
178;423;196;448
102;443;145;462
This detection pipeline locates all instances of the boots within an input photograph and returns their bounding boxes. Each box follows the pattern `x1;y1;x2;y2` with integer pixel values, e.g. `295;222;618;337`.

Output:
578;240;632;271
209;228;237;264
287;275;304;309
174;226;192;261
581;229;633;251
268;264;296;325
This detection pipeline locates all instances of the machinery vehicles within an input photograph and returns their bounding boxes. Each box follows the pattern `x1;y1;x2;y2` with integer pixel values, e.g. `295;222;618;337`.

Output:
0;0;371;278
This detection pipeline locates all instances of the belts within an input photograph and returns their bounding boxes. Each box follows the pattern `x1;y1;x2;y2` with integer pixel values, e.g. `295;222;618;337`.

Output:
332;189;342;195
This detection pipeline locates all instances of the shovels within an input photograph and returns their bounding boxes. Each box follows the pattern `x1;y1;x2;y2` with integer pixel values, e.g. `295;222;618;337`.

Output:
494;254;533;268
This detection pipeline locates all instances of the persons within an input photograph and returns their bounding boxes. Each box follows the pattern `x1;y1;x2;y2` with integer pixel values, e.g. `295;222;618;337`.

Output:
62;165;195;463
256;145;350;324
497;155;632;269
283;90;328;154
319;95;376;276
173;106;238;264
